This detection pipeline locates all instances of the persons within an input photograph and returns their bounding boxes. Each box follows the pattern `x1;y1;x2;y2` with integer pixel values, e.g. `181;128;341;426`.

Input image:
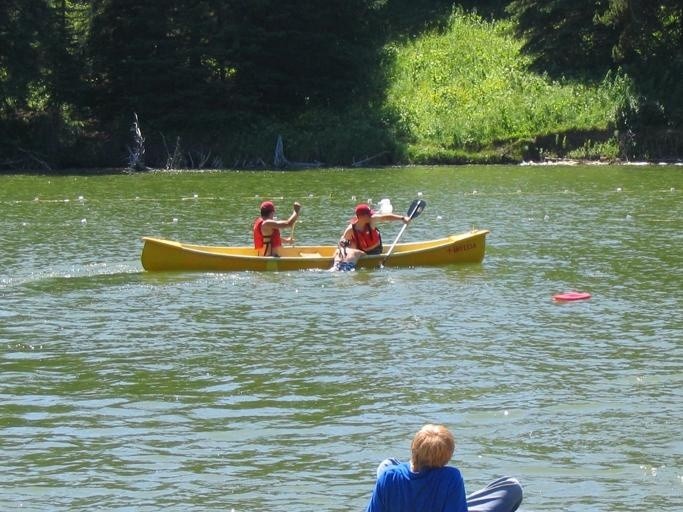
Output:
363;423;523;512
340;204;411;254
253;201;300;257
327;238;366;273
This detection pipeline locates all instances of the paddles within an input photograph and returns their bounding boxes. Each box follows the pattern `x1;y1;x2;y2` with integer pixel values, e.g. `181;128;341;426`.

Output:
380;200;426;269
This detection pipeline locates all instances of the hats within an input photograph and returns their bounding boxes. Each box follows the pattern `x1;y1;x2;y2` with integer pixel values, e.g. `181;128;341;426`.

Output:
261;201;275;212
355;204;375;215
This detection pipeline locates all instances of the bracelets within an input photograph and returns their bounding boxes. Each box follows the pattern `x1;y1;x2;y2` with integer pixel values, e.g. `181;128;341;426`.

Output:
400;215;404;221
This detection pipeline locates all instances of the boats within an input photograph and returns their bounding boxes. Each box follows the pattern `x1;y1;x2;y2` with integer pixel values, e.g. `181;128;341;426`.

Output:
141;229;491;272
